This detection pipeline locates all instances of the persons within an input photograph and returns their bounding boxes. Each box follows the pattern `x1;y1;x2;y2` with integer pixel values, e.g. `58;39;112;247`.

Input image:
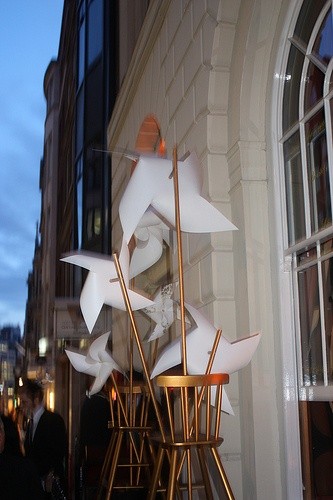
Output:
20;378;66;500
74;369;172;500
0;392;41;500
